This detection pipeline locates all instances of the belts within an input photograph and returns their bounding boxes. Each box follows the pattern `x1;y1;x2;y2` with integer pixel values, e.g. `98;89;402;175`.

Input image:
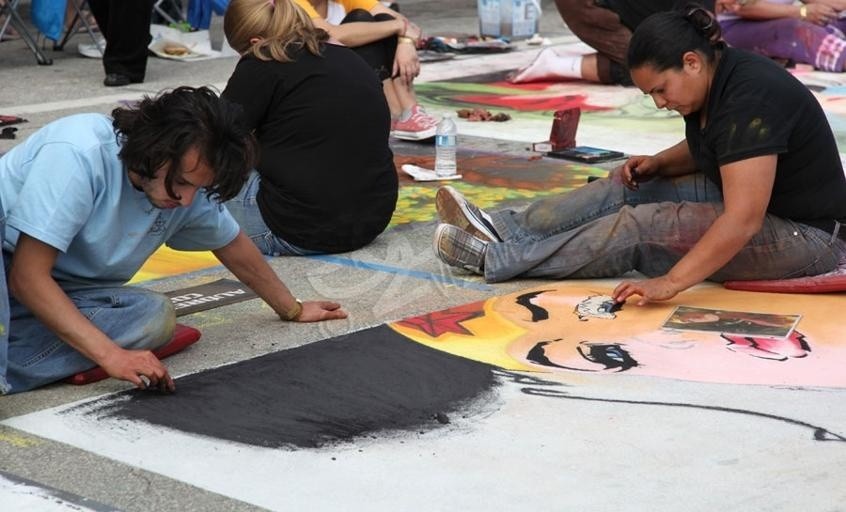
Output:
789;219;846;237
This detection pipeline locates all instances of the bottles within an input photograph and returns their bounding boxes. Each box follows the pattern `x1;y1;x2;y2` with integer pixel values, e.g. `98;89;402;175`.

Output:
434;111;459;179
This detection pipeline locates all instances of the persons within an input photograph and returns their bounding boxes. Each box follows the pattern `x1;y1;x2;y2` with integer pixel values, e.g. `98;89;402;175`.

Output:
218;0;399;256
434;0;846;309
89;0;153;87
1;85;351;398
505;0;714;89
286;1;443;142
716;0;846;80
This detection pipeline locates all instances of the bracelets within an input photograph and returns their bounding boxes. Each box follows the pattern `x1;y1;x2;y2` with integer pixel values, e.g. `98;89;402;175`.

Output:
401;19;409;38
798;6;808;20
281;296;305;321
397;38;417;46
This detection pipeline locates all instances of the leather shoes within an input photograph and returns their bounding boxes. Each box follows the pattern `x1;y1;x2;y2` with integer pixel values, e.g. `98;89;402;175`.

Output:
104;74;131;85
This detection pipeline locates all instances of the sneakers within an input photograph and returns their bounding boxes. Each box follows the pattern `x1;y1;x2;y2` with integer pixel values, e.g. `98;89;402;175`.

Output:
389;115;436;140
77;41;107;58
413;106;440;130
433;223;489;276
436;185;504;243
